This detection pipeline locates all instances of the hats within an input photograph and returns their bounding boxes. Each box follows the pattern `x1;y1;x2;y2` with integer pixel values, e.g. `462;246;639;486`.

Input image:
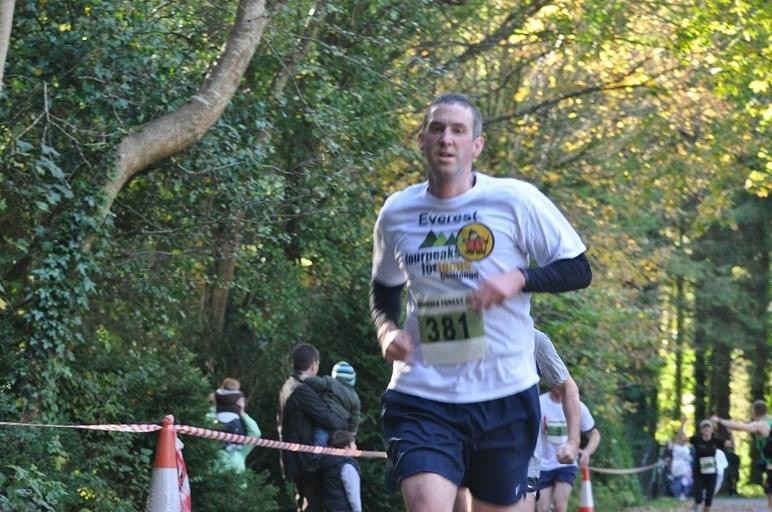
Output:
332;360;356;387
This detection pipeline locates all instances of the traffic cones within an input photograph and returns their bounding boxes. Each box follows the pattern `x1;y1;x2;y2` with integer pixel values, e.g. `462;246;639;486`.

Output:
578;464;596;512
145;416;182;512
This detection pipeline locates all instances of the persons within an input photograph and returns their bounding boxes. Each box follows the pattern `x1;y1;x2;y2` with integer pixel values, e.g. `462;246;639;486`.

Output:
214;377;245;450
322;427;363;512
654;430;739;502
536;385;601;512
676;415;736;511
710;399;772;512
276;342;353;512
298;360;360;473
452;326;583;510
204;392;264;489
367;91;594;511
